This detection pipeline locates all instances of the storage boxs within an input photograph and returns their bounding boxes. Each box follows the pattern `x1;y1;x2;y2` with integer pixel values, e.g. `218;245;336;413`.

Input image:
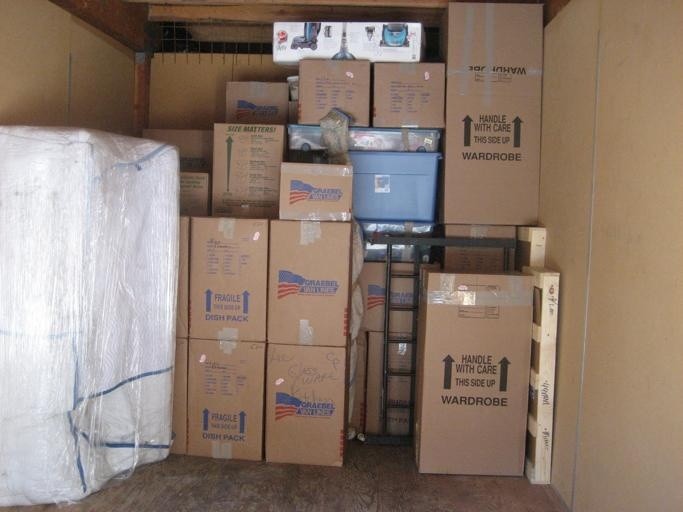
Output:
141;123;442;468
224;79;289;126
434;2;543;228
287;99;298;126
437;219;519;279
369;61;445;132
287;76;299;101
411;260;533;477
297;58;371;129
271;20;428;68
